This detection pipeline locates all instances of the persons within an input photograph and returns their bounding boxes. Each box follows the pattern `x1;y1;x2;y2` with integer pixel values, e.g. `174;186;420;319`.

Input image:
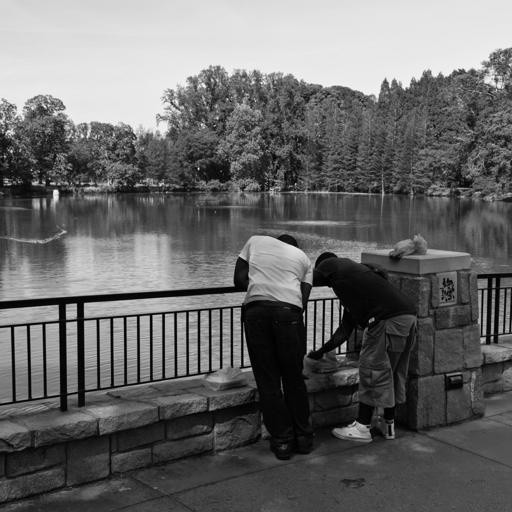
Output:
308;249;420;444
233;232;318;460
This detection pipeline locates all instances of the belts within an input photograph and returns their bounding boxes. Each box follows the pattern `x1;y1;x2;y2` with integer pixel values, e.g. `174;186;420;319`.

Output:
242;300;302;313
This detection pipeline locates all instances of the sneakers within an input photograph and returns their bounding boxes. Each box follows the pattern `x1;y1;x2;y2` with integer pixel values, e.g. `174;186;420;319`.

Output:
291;436;313;455
269;441;292;461
331;420;373;444
375;418;396;440
